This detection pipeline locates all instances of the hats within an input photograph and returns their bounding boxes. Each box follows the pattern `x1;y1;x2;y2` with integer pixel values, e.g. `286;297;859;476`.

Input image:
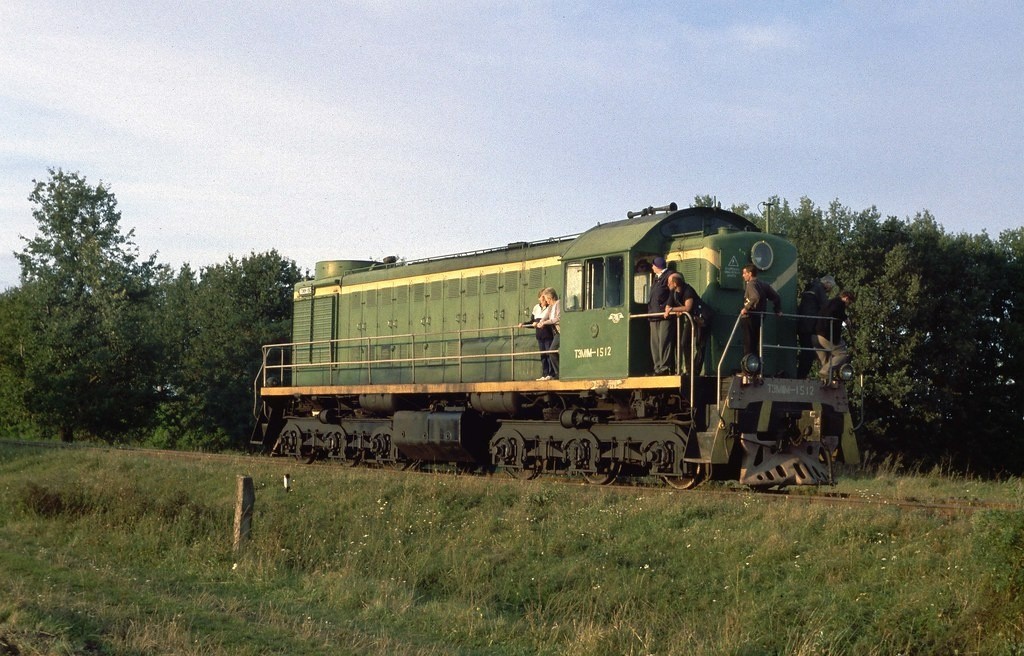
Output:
652;256;666;269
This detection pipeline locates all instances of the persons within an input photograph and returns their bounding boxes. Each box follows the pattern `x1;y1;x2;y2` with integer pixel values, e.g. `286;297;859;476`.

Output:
647;257;674;375
519;289;550;380
664;272;713;376
795;274;835;380
816;291;857;382
736;265;783;378
537;287;560;380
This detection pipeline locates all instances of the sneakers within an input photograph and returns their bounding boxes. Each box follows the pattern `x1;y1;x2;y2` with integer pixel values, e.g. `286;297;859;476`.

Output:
536;377;546;381
543;375;555;380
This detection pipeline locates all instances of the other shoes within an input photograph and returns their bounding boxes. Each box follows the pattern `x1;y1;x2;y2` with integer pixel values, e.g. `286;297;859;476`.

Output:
645;370;654;376
652;370;669;376
735;372;749;377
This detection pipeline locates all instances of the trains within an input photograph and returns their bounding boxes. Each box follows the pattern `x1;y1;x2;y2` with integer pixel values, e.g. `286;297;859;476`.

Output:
250;202;866;493
